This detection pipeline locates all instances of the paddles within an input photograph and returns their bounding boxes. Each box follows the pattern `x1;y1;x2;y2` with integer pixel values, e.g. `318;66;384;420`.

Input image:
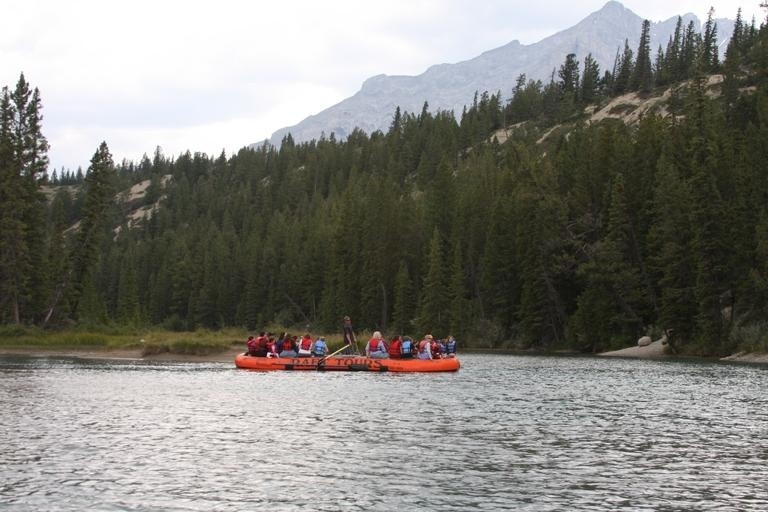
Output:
318;345;350;365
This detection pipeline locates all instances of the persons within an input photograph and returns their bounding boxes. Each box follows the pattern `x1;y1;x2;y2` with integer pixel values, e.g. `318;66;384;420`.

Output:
246;331;330;358
343;315;357;356
365;330;456;360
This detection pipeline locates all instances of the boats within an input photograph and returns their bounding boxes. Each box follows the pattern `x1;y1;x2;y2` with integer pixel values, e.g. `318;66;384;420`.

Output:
235;352;459;372
